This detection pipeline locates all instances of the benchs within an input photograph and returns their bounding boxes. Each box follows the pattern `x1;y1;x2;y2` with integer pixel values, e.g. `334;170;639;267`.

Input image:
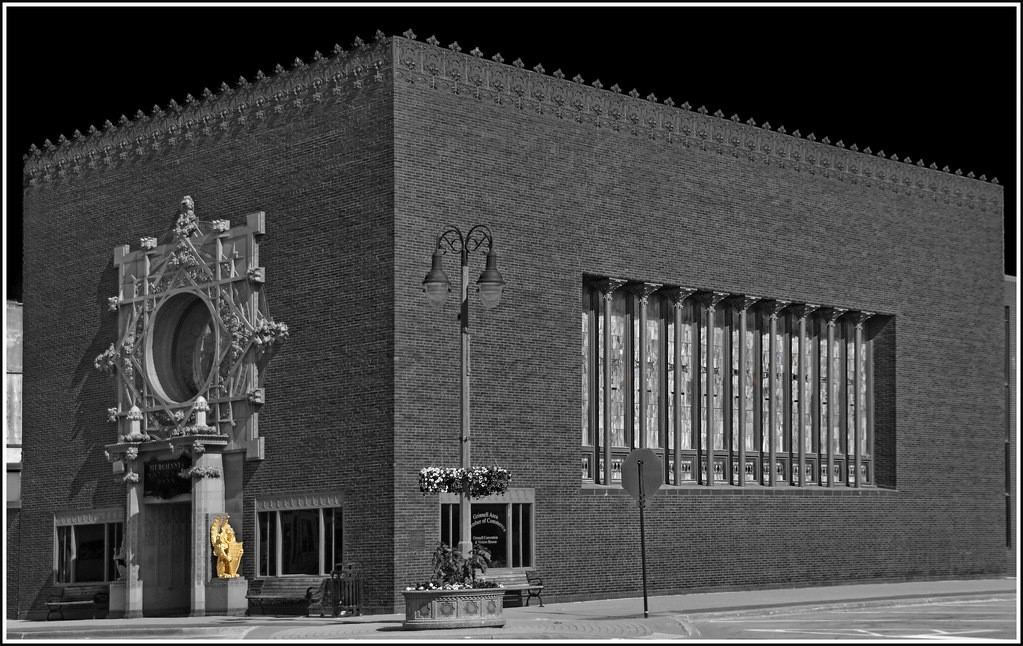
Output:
242;573;344;617
475;566;544;609
43;586;109;621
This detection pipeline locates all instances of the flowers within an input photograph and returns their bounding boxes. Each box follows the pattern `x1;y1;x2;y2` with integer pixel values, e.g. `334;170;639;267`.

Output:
418;465;511;498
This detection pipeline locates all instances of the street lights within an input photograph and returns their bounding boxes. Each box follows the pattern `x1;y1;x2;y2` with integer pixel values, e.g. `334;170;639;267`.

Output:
422;221;505;589
